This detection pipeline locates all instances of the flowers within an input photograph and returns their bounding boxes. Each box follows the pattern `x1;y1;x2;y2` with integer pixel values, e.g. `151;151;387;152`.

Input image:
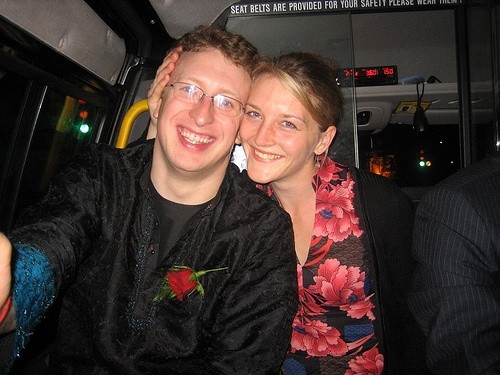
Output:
151;264;228;302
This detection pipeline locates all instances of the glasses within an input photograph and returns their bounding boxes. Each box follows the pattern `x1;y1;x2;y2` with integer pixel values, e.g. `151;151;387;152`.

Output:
165;82;245;117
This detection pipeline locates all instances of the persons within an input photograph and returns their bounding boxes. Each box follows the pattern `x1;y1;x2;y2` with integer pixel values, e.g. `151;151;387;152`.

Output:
0;25;300;375
405;152;500;375
146;44;415;375
